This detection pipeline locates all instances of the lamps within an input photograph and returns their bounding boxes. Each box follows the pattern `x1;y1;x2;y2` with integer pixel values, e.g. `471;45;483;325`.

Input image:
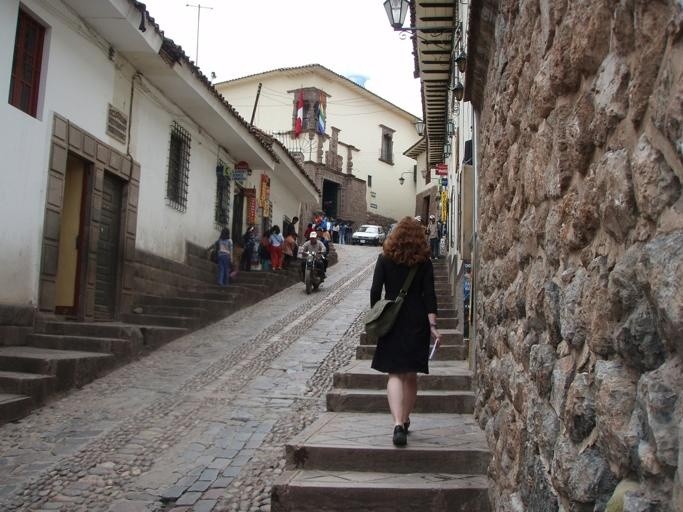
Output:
421;168;438;179
398;172;414;186
383;0;462;30
413;121;425;136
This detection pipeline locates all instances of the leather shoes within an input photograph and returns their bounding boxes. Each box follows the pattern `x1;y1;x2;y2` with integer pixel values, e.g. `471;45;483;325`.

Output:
393;421;410;447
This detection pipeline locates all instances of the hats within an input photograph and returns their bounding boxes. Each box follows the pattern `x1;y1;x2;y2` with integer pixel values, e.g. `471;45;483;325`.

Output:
430;215;435;219
309;231;317;238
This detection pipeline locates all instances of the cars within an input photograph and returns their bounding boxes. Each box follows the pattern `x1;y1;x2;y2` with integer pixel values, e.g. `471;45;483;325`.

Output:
351;223;385;246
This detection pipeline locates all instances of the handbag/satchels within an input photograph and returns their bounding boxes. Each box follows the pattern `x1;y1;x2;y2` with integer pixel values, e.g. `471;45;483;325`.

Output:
211;251;217;262
365;299;403;336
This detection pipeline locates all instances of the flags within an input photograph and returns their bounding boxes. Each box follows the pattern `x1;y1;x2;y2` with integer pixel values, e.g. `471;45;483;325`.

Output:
316;90;327;135
295;88;304;137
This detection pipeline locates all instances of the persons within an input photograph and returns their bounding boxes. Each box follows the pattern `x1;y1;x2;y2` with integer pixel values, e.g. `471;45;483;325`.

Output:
203;211;353;287
362;214;443;448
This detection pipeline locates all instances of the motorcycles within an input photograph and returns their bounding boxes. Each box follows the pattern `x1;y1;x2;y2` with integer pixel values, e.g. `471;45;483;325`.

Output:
298;248;330;294
